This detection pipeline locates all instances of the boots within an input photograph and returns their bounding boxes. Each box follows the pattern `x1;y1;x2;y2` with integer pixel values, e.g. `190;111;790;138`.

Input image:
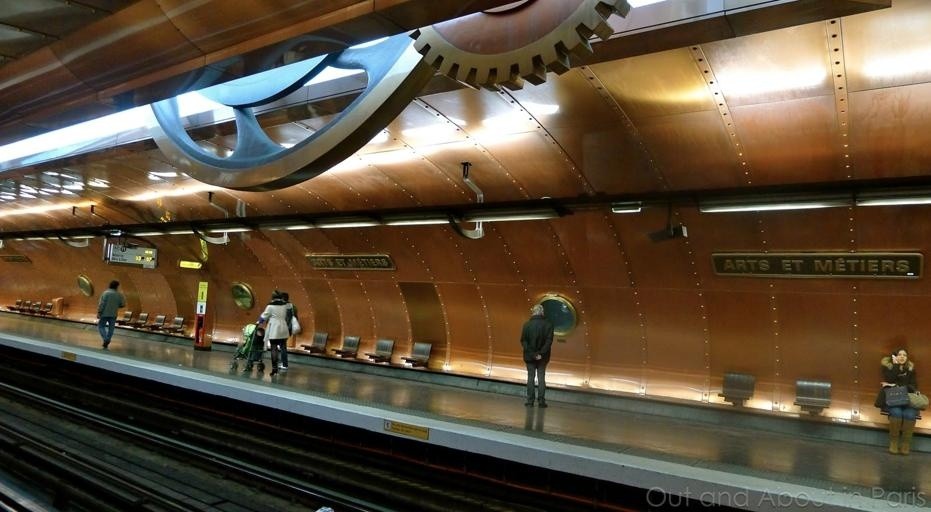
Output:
887;416;916;455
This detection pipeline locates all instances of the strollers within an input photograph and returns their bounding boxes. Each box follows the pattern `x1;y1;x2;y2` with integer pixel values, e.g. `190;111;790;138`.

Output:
229;323;266;373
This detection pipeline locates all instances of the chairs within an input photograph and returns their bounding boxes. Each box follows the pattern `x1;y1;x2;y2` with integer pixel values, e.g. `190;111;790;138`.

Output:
116;311;184;335
7;299;53;318
300;331;432;368
717;372;756;407
792;379;832;416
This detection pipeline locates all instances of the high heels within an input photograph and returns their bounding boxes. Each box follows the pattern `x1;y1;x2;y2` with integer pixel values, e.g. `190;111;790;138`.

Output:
270;369;277;375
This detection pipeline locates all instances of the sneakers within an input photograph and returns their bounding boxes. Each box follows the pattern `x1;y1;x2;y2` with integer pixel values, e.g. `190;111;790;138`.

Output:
524;403;547;408
278;362;288;370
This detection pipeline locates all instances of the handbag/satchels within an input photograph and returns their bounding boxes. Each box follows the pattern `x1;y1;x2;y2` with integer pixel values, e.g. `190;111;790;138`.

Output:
908;392;929;410
291;316;301;335
885;385;910;407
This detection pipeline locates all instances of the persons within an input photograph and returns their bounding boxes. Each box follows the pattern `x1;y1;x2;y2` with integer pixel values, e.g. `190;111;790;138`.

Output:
256;290;293;376
96;281;125;348
278;293;298;369
874;347;920;456
520;304;554;408
